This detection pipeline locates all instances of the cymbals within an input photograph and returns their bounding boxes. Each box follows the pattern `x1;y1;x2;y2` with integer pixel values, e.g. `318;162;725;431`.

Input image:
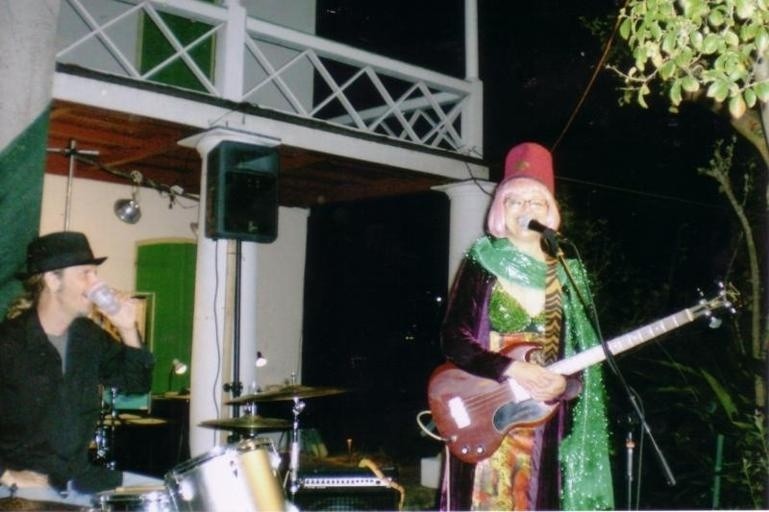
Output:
224;385;350;404
199;417;292;428
1;497;94;511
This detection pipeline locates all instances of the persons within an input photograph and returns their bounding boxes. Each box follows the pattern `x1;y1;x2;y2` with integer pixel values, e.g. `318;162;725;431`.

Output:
1;228;154;491
438;174;588;510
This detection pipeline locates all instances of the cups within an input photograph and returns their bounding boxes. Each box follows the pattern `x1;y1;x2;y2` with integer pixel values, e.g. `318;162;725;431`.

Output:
87;281;121;316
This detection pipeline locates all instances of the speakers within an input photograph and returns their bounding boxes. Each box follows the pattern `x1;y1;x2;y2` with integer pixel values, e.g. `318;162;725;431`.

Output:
205;140;282;244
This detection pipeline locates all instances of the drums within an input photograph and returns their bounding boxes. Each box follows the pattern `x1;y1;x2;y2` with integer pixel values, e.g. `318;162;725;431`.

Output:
94;486;167;511
167;435;291;510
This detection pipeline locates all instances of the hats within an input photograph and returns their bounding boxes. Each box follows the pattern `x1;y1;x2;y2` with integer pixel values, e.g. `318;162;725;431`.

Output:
495;142;554;194
14;230;108;281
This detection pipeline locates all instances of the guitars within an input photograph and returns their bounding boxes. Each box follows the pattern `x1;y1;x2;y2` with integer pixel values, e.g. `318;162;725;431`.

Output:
427;279;744;463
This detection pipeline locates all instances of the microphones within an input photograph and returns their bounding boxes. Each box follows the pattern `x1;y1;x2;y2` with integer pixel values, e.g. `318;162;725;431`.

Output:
514;211;562;243
